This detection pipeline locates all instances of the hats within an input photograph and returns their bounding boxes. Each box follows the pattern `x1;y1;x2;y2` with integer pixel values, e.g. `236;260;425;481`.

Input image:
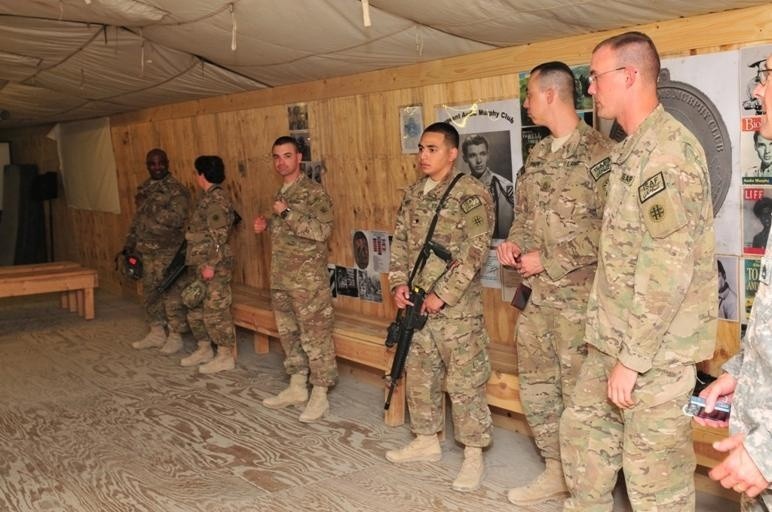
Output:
181;273;207;309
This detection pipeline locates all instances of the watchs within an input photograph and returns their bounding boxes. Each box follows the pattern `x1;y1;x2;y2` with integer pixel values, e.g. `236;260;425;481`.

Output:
280;207;288;218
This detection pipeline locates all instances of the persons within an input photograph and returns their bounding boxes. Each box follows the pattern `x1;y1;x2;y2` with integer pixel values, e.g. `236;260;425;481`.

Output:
117;148;192;357
751;195;770;248
746;54;765;114
353;231;369;270
685;51;771;511
404;116;420;147
180;154;241;372
328;265;348;297
382;123;496;490
251;135;337;424
557;31;716;511
461;136;513;237
359;277;382;302
716;260;739;317
499;60;622;506
290;105;322;182
750;130;772;181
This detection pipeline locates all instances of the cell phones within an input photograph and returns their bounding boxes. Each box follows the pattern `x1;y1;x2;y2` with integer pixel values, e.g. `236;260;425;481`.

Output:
685;396;731;423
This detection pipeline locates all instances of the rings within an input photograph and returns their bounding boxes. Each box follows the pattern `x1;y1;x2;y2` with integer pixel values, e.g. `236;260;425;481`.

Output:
736;483;745;491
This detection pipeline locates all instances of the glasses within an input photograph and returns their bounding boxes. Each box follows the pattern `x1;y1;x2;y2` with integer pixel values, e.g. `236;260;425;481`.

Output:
757;66;772;86
588;66;637;84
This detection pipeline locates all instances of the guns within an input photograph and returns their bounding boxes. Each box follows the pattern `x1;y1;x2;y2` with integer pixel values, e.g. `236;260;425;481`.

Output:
143;209;242;309
384;286;446;409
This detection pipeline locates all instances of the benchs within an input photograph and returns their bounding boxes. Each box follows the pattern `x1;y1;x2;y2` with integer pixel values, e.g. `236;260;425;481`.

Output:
1;261;99;320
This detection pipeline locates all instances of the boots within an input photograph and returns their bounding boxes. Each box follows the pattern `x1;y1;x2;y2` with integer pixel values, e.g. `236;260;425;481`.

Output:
180;340;214;367
298;385;329;423
385;433;441;463
507;459;571;506
263;374;309;410
452;446;485;492
159;332;184;354
198;346;235;374
132;326;167;349
